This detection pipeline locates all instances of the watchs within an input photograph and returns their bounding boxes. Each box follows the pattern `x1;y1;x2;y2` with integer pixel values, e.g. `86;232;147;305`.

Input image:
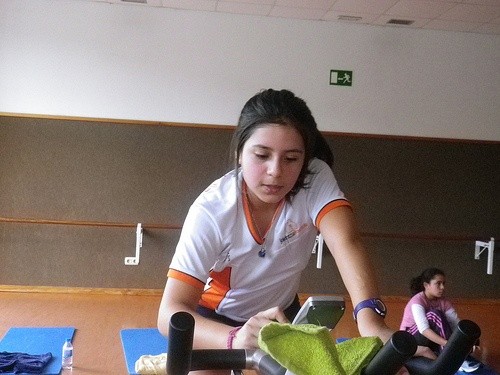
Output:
352;298;388;323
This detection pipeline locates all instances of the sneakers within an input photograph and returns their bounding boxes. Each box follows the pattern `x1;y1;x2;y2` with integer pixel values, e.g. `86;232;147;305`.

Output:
458;358;481;373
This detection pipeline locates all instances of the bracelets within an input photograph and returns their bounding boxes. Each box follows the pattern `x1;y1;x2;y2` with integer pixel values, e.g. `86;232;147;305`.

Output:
227;324;241;349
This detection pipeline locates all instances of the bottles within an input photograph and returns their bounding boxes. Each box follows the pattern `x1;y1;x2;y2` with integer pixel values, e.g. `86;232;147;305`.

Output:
62;339;73;371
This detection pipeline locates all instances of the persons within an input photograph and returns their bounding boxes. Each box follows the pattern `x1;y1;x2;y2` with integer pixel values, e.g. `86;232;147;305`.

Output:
397;266;483;373
157;88;440;375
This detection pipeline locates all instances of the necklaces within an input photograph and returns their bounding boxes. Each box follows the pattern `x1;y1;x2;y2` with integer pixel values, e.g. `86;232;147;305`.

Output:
247;194;274;257
